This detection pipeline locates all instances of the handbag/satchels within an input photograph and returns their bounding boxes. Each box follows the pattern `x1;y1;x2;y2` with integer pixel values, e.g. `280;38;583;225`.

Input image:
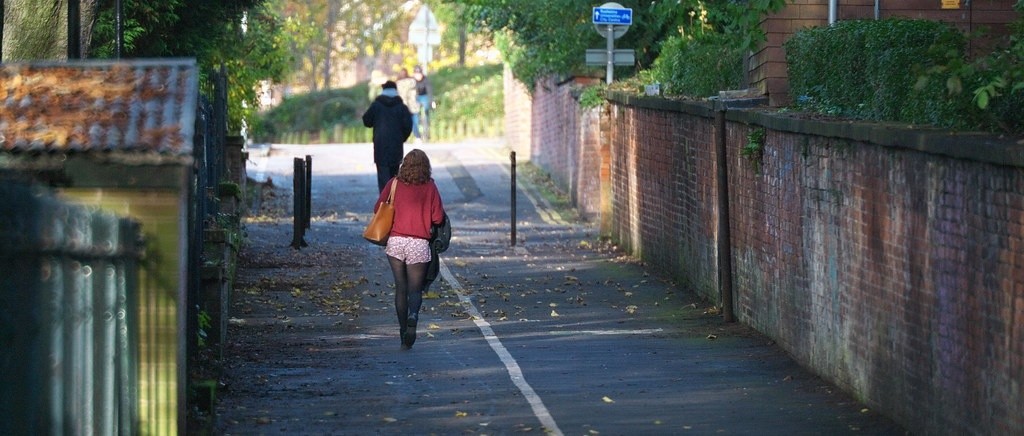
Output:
362;176;398;246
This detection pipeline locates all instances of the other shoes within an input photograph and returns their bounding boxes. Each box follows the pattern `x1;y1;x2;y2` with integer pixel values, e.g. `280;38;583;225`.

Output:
400;328;406;344
405;313;418;346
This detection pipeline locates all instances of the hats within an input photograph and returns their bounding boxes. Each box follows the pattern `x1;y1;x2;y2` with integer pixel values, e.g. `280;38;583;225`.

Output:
382;80;396;90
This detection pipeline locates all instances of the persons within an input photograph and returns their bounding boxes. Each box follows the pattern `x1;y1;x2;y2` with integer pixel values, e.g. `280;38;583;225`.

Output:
362;81;413;195
396;65;436;144
373;149;444;348
